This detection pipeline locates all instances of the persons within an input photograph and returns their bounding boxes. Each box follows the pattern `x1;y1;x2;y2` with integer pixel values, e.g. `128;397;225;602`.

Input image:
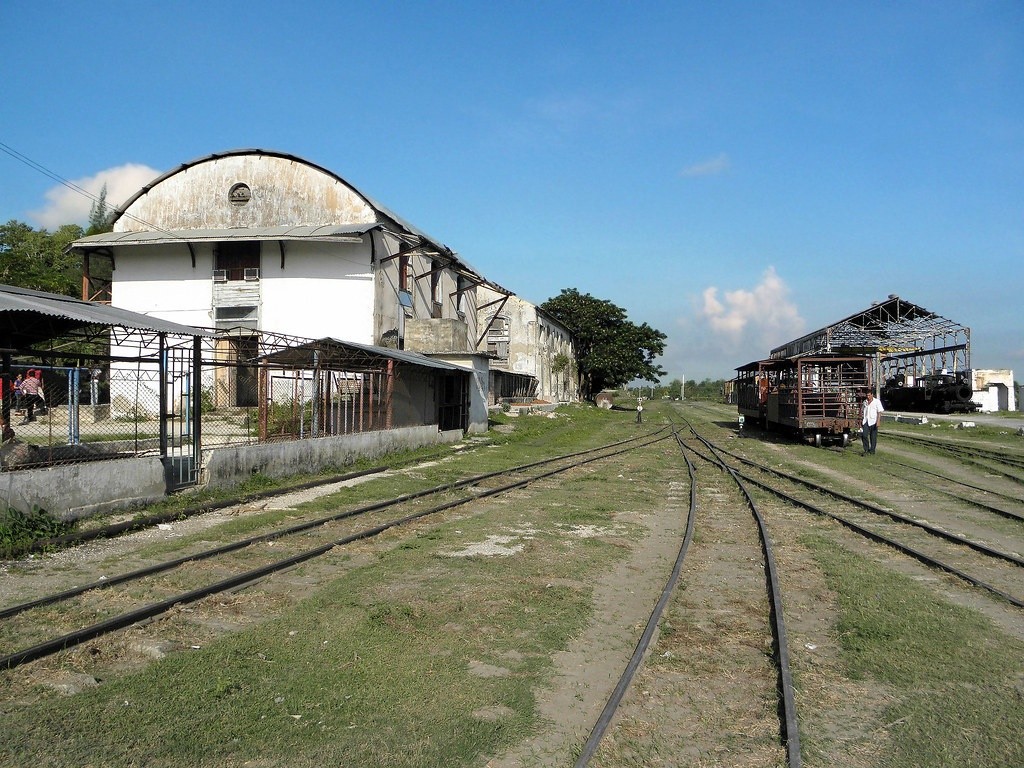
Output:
859;390;884;456
26;362;49;411
19;371;47;422
14;373;23;416
0;377;14;413
88;363;102;407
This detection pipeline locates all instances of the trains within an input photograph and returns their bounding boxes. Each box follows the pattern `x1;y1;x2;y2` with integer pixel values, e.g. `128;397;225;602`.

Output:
874;370;976;415
733;348;874;448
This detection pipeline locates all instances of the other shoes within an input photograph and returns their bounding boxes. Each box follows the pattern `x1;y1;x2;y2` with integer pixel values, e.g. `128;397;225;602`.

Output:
863;450;870;456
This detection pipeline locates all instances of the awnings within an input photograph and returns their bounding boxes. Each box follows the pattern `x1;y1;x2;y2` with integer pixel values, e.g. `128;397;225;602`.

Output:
0;283;224;340
